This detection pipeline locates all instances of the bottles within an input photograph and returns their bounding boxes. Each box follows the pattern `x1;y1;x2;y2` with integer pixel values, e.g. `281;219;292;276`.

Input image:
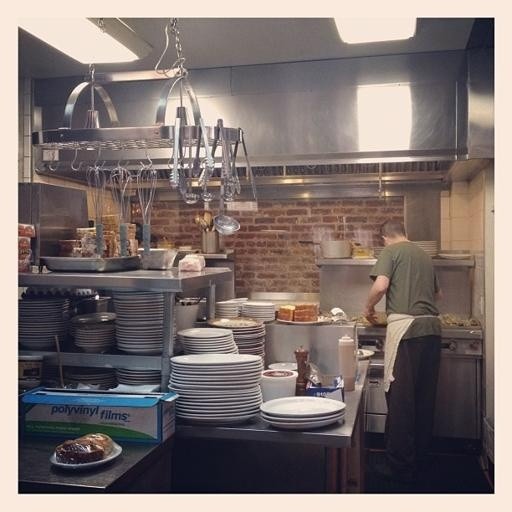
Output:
337;334;358;393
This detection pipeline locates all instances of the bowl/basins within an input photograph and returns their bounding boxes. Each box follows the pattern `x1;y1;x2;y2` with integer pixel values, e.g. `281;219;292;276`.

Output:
137;247;179;270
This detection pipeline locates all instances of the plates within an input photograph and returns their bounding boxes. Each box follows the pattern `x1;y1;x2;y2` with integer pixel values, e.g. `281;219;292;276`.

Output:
48;442;124;472
167;313;349;431
410;240;472;260
18;290;177;392
214;297;333;324
355;348;375;360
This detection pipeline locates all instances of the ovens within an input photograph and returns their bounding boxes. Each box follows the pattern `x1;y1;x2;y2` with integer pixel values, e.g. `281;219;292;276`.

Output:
358;336;482;440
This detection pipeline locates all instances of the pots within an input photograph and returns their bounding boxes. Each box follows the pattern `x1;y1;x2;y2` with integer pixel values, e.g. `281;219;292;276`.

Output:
296;239;352;259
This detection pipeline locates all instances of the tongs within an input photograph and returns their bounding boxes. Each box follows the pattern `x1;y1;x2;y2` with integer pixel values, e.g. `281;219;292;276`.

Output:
192;116;216;179
208;118;233;183
233;126;258;199
168;116;184;191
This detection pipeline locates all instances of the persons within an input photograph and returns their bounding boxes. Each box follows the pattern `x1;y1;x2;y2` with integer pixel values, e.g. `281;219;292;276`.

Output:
361;217;444;484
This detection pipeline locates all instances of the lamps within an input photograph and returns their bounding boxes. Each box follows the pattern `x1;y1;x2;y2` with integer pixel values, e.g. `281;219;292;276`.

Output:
18;17;154;65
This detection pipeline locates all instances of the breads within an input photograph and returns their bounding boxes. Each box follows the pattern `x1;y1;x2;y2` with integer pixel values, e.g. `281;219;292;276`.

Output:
278;302;320;322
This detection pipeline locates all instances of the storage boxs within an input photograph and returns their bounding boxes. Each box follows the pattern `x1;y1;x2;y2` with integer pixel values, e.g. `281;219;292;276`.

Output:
19;386;180;444
